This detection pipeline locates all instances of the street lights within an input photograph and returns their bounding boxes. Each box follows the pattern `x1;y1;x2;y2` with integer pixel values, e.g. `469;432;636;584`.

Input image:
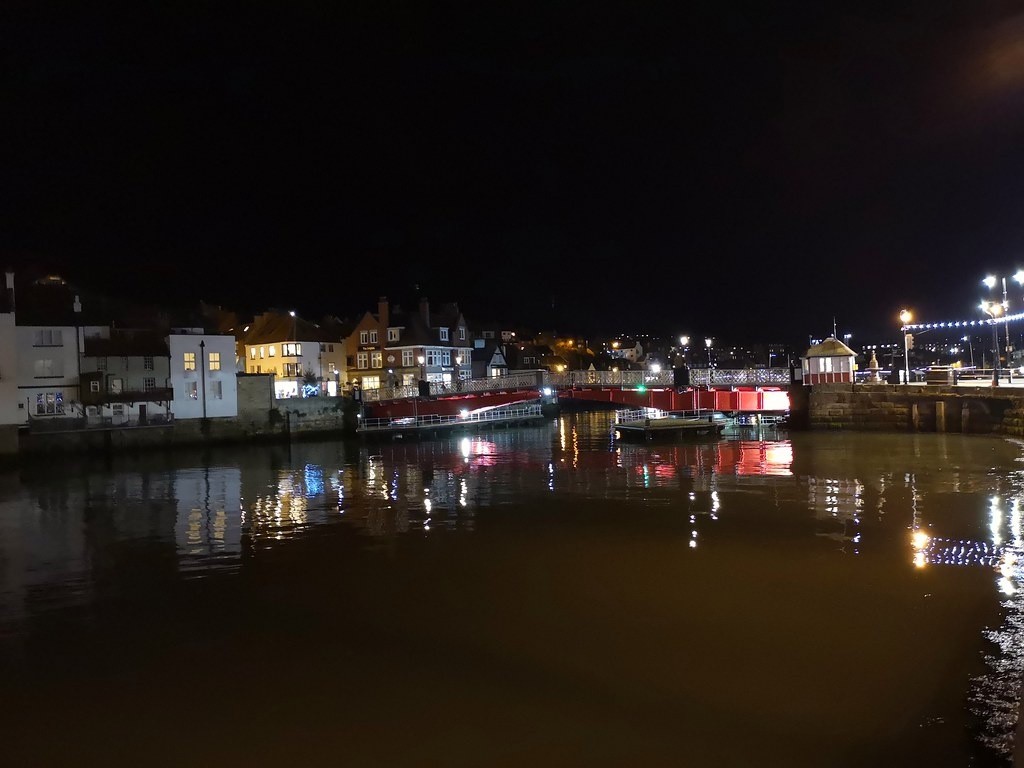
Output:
388;369;396;398
333;370;339;396
680;336;688;369
418;356;425;380
899;309;913;383
983;270;1024;370
978;299;1009;379
705;340;712;368
456;357;463;379
